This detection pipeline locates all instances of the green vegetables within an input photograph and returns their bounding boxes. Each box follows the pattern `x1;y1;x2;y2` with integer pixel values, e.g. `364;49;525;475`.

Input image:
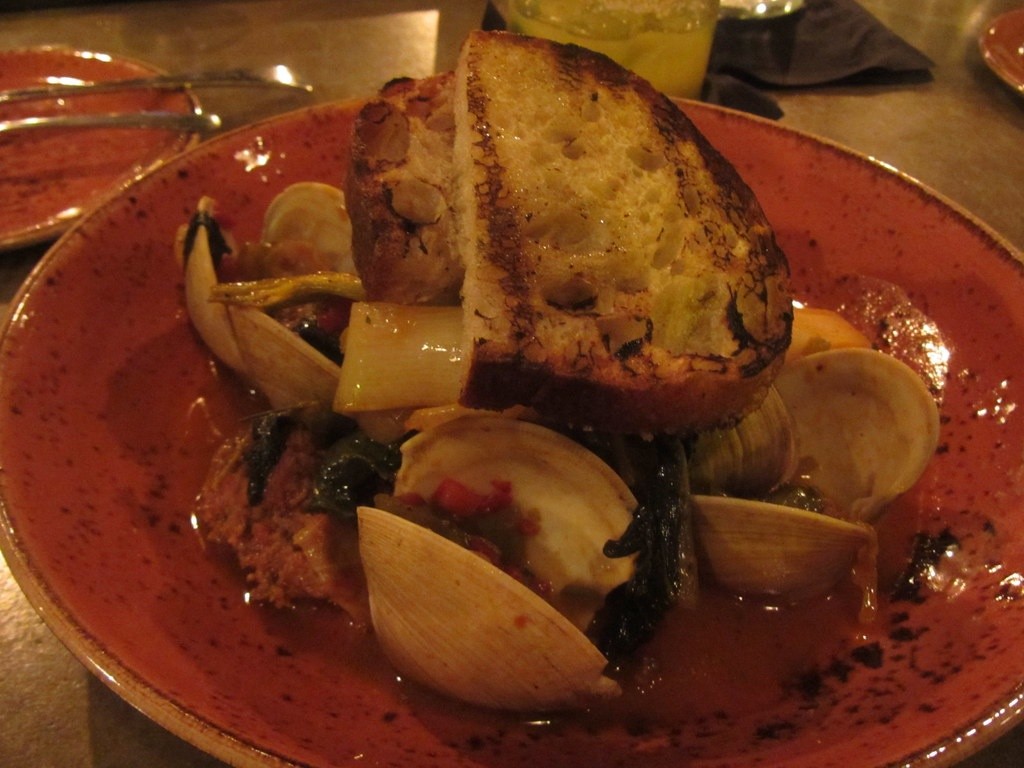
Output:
180;209;687;677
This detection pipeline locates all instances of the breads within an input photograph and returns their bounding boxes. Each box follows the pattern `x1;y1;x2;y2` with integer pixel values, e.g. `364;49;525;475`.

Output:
345;70;464;302
456;28;792;438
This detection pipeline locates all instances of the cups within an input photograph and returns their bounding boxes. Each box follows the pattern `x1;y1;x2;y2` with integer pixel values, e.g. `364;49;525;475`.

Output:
507;0;720;102
708;0;807;82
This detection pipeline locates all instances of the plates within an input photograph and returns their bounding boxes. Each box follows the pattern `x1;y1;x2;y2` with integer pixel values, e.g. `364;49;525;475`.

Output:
0;85;1024;768
0;43;204;250
979;8;1024;99
